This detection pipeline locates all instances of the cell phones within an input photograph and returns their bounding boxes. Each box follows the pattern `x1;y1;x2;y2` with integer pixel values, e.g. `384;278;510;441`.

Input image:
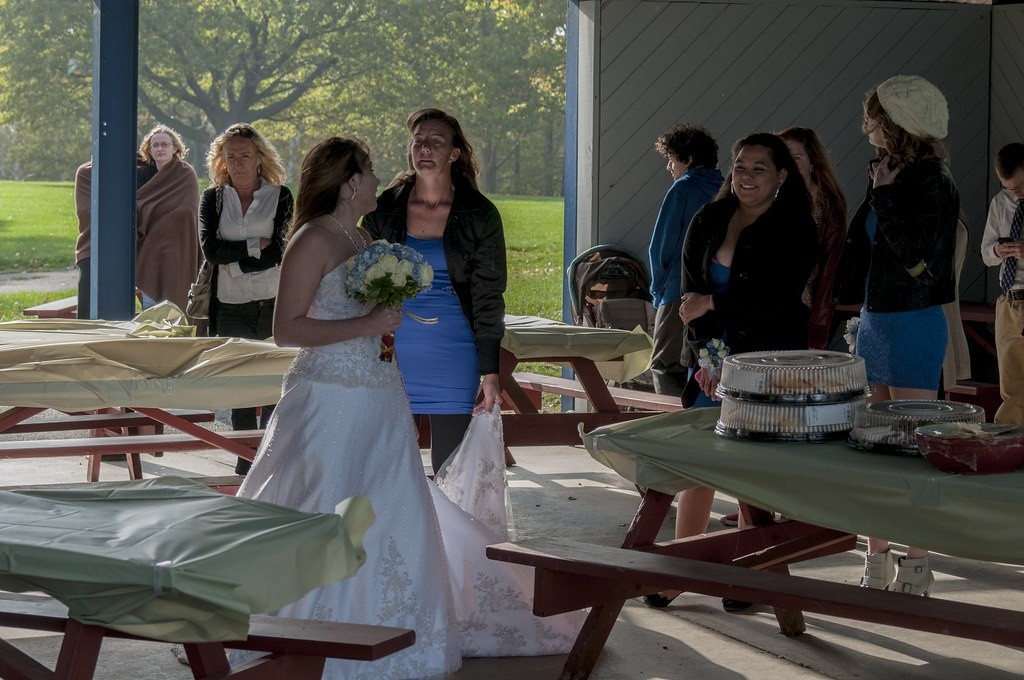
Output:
998;237;1014;244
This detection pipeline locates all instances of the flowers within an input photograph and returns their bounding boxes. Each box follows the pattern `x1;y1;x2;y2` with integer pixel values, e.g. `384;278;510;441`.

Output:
344;237;435;312
698;336;731;382
843;317;861;354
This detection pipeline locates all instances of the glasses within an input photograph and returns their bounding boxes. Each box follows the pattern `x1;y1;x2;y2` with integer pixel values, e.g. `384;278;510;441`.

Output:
149;142;175;149
228;127;253;138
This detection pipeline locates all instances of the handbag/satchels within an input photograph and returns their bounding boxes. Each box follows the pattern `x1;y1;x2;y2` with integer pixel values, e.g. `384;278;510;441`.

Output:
185;260;214;319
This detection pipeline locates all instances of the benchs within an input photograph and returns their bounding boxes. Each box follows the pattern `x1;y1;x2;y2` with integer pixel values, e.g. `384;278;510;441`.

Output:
23;294;78;322
944;379;999;396
0;600;414;680
511;371;686;412
0;429;265;463
484;537;1024;650
0;410;215;458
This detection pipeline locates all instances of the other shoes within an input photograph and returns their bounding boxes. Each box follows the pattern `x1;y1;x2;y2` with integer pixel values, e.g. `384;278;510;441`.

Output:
721;514;739;526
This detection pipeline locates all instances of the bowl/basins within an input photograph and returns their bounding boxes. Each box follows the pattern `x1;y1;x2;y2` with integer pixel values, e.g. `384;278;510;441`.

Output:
914;422;1024;475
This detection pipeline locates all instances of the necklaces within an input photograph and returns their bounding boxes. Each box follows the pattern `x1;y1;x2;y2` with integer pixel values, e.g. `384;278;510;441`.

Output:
325;215;369;254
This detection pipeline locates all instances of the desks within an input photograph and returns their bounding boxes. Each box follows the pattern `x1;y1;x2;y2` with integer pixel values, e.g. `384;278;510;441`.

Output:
0;316;1024;680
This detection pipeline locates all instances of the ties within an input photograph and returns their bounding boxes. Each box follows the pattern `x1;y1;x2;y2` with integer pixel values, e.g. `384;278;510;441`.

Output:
1000;198;1024;291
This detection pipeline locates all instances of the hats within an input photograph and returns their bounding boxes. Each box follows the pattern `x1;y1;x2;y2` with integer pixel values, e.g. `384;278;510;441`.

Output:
876;74;949;141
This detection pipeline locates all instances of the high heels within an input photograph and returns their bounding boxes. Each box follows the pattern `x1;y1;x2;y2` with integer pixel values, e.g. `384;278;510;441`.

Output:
642;588;686;607
887;555;935;599
722;597;752;612
860;549;898;591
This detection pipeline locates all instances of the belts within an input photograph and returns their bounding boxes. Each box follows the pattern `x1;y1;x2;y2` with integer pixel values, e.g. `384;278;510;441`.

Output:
1003;289;1024;301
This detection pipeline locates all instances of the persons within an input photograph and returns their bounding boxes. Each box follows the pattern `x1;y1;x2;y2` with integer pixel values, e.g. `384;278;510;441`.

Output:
75;124;201;325
360;109;507;486
640;75;1024;610
243;136;464;680
198;122;295;474
